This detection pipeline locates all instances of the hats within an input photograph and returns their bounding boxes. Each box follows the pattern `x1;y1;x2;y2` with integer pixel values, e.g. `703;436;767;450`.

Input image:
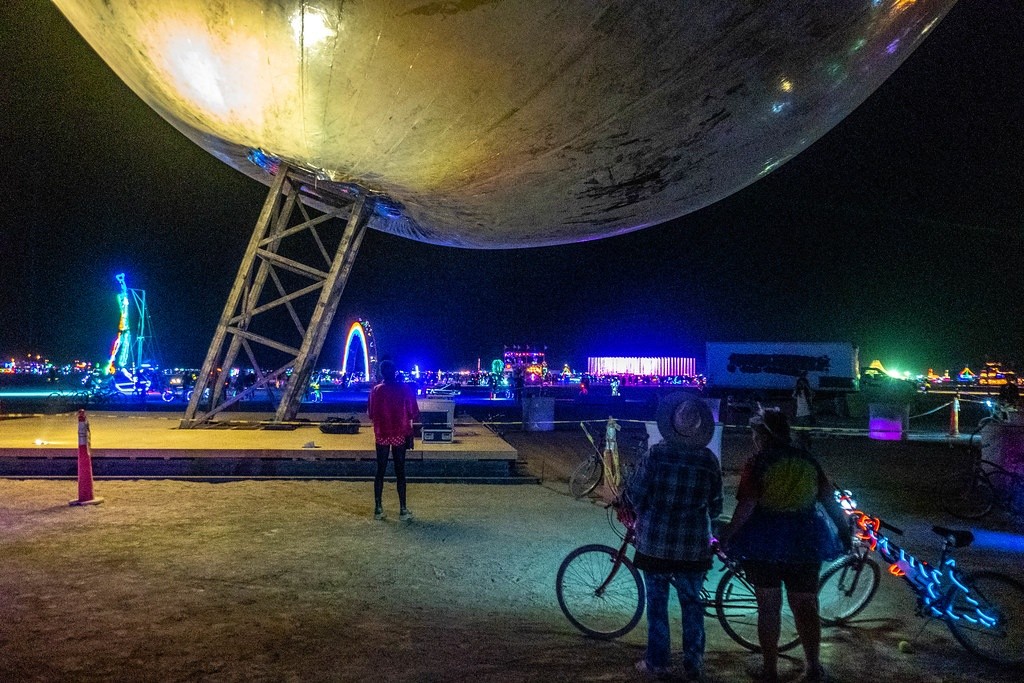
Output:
655;393;716;450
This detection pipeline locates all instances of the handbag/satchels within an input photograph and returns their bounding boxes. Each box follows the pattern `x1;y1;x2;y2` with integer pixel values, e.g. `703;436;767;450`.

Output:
404;435;415;450
808;502;845;560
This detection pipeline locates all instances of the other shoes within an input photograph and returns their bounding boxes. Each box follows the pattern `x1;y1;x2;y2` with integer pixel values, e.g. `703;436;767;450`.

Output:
802;664;824;683
374;507;386;521
747;667;779;683
637;661;672;675
399;510;412;520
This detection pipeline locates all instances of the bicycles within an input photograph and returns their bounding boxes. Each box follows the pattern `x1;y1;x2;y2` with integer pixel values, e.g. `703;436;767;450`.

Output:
45;381;323;411
816;479;1024;671
553;487;800;654
567;422;651;496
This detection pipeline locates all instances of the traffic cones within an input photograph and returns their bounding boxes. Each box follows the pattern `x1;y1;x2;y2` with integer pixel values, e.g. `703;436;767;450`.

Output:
945;396;962;439
67;408;105;505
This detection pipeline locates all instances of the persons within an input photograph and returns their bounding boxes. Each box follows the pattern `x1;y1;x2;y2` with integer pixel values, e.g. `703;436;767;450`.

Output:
48;366;56;385
999;374;1021;420
182;371;229;401
84;366;151;395
575;384;590;419
245;371;256;399
617;391;724;678
369;360;414;521
925;379;931;390
721;407;850;682
791;378;813;427
